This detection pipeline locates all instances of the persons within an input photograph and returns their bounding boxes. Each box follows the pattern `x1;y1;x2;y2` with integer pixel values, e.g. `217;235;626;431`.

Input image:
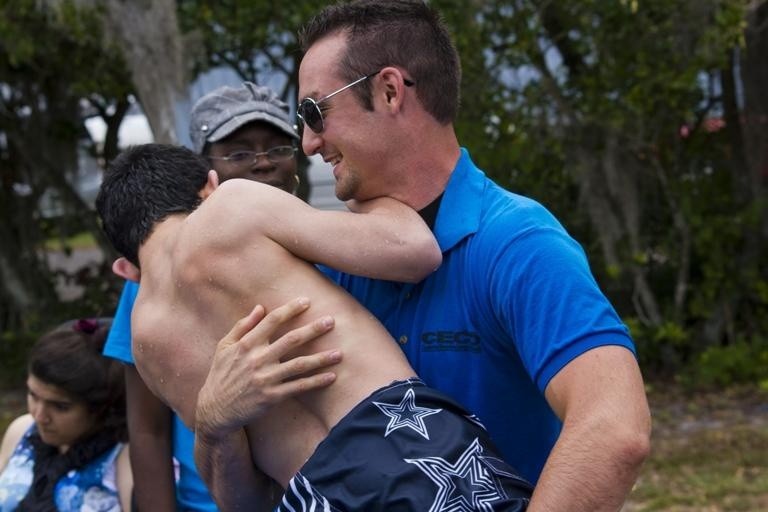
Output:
186;0;657;512
96;80;315;508
90;140;550;512
0;314;157;511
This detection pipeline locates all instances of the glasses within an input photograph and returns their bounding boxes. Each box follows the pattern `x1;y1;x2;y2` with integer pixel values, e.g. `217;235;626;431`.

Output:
204;146;300;166
296;68;414;136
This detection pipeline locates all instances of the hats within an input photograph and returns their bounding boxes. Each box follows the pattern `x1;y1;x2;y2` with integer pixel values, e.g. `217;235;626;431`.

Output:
187;79;298;159
